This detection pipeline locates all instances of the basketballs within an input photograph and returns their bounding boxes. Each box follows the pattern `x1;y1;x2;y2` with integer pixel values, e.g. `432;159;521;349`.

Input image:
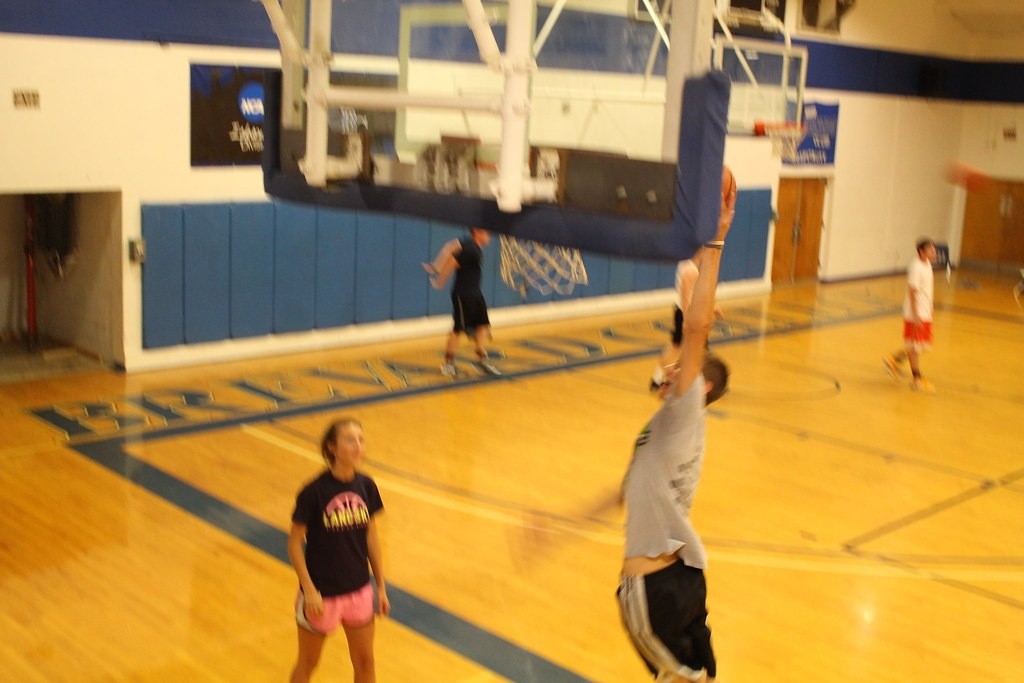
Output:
721;166;736;207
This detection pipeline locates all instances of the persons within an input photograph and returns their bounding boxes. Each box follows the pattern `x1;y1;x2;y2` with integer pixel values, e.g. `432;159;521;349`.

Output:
422;227;505;378
516;190;735;683
287;418;391;682
650;243;724;389
883;236;936;391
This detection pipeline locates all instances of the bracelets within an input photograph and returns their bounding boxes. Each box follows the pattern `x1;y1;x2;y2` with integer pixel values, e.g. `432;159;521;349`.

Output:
702;240;725;250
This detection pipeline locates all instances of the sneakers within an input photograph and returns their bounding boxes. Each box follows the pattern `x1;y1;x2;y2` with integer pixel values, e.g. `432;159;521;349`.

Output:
909;380;936;394
440;360;457;377
882;354;908;387
649;376;665;393
471;360;502;376
